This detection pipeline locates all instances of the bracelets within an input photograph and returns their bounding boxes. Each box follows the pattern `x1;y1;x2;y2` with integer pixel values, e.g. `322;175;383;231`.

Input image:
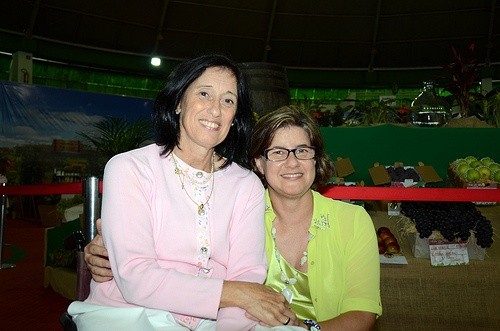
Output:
304;319;321;331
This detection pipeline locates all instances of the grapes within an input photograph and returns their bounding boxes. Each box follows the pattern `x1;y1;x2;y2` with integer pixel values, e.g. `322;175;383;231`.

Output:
387;166;420;182
401;200;494;248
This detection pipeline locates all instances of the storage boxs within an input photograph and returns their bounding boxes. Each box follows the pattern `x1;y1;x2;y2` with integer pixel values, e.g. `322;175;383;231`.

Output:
369;166;443;211
408;230;486;261
61;203;83;223
311;158;364;207
448;162;498;206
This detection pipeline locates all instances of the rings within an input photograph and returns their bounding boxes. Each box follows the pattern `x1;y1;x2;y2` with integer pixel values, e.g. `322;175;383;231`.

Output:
283;316;291;325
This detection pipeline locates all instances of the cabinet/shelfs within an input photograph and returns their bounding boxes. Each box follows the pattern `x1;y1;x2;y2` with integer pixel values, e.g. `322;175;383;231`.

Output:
290;89;500;331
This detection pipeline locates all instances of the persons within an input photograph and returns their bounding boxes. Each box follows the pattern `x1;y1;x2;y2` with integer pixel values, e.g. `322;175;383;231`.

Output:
68;55;310;331
83;103;383;331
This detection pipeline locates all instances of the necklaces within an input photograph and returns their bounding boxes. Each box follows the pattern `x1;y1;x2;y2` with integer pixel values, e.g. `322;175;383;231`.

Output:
271;222;309;306
171;150;215;216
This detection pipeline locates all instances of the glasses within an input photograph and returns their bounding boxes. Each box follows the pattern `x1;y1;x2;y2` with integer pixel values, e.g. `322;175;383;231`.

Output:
260;145;316;162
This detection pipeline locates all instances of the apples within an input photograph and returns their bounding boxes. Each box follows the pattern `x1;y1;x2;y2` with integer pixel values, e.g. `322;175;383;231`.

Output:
376;227;400;255
